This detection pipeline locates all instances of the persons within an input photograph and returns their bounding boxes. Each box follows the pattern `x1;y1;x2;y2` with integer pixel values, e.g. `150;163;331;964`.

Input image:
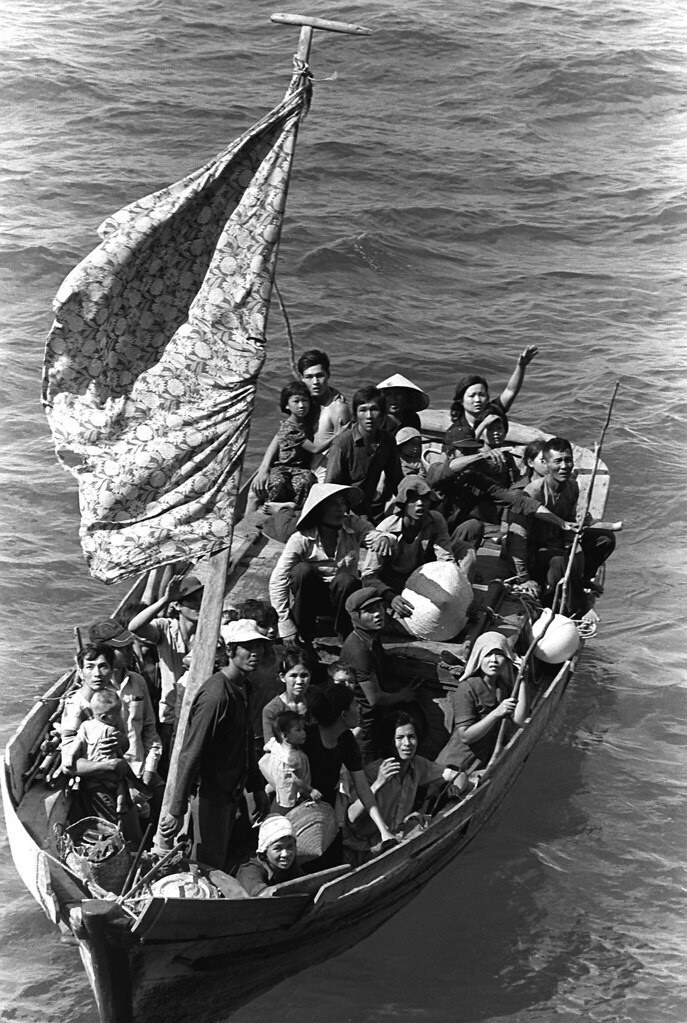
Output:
56;346;615;899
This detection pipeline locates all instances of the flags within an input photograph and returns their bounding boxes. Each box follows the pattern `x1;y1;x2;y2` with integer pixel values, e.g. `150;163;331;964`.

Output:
40;57;311;581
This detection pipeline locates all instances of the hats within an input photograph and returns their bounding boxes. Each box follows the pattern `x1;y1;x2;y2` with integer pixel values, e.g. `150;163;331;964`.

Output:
394;426;422;445
88;619;137;648
401;561;473;642
394;474;446;510
296;480;365;531
172;575;205;601
284;800;340;866
375;373;431;413
221;619;274;644
345;586;384;612
255;813;296;853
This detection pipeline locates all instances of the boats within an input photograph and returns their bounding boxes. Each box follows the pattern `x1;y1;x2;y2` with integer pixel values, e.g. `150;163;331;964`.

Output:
0;12;621;1023
